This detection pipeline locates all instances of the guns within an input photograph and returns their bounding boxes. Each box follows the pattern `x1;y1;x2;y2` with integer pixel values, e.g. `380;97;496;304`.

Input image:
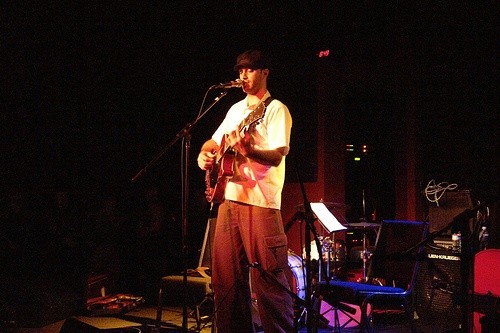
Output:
341;222;382;228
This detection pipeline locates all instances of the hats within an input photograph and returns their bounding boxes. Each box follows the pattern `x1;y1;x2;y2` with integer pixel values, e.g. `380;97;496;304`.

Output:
234;49;269;71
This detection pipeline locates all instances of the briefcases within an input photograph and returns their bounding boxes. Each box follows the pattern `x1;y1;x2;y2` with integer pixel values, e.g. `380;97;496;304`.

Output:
81;271;146;316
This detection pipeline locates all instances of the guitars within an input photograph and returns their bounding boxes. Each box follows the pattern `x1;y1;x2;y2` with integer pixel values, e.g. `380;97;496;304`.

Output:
204;101;266;205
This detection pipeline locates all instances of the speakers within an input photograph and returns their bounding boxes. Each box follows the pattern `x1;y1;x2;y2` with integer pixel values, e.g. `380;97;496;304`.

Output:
410;252;464;333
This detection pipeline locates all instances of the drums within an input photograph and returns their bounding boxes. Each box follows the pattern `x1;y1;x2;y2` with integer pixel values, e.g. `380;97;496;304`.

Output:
301;235;349;273
349;244;379;270
248;247;310;328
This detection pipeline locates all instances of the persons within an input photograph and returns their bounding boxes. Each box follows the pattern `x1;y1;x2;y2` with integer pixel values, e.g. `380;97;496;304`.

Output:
197;50;294;333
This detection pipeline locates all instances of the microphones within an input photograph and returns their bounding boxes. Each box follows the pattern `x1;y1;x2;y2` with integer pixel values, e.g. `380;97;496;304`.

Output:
214;79;243;87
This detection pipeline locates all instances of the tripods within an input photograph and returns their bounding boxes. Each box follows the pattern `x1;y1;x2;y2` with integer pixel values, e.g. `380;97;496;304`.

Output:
283;138;341;333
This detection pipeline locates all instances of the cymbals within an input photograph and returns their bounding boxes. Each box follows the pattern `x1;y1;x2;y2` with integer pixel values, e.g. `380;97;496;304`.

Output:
292;202;342;211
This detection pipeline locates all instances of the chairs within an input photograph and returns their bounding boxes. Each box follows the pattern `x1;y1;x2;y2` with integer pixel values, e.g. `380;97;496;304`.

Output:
310;218;429;333
156;218;217;333
